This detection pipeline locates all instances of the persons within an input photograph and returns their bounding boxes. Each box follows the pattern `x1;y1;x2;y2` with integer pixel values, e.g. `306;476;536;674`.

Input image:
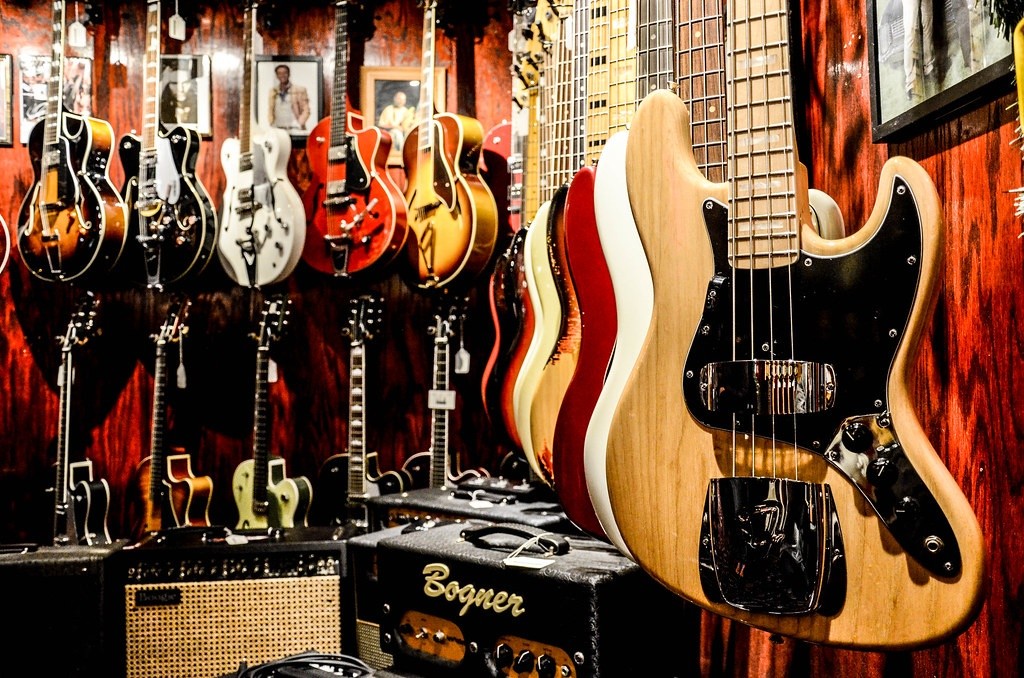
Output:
268;65;311;133
159;59;198;125
379;92;415;152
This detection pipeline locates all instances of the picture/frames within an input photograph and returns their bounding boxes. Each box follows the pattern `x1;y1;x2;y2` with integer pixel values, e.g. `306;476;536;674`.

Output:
18;54;93;146
359;66;447;168
0;53;14;147
141;53;214;139
253;53;324;142
865;0;1017;146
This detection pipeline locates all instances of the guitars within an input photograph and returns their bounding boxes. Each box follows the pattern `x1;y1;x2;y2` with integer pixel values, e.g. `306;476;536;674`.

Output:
18;0;129;283
26;289;111;548
315;295;411;525
302;0;410;280
211;0;306;288
402;289;492;493
476;0;983;649
401;0;500;291
117;0;219;292
131;293;213;541
231;291;314;530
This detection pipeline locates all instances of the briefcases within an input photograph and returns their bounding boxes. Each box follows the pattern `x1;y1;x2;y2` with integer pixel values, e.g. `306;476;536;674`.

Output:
0;538;129;678
